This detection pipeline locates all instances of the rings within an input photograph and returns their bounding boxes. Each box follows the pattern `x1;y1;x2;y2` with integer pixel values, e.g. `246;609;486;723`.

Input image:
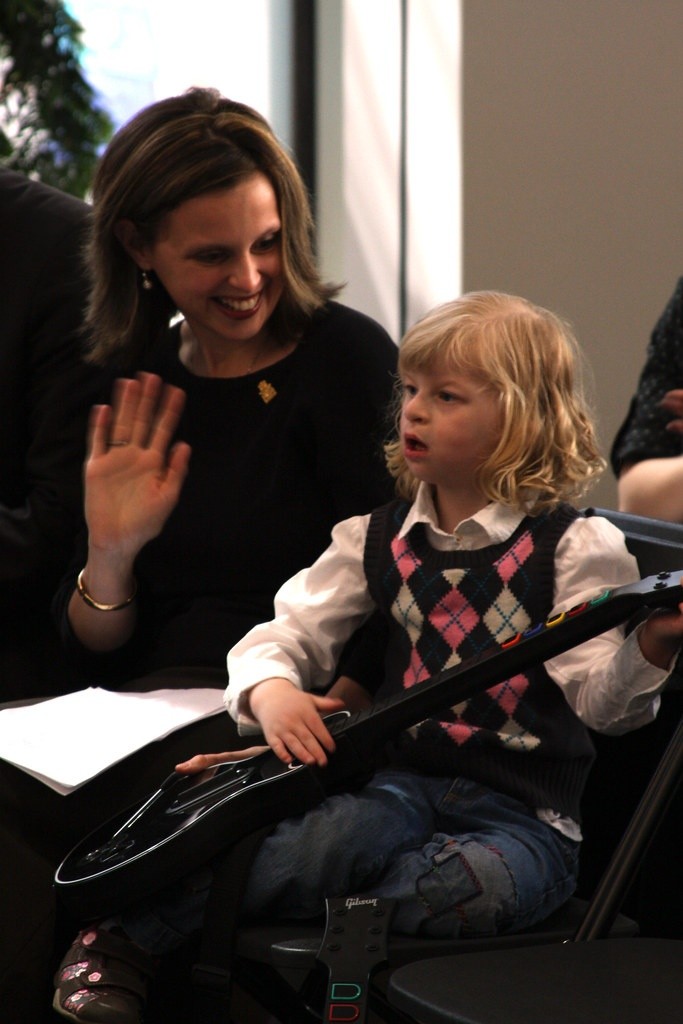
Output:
107;438;129;447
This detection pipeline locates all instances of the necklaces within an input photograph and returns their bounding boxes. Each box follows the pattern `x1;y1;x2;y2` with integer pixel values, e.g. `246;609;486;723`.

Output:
183;320;275;375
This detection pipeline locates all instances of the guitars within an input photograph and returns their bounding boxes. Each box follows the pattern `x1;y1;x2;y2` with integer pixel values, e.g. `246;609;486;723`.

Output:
54;571;683;924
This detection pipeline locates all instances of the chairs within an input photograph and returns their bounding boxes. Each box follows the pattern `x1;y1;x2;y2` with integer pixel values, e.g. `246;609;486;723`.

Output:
269;506;683;955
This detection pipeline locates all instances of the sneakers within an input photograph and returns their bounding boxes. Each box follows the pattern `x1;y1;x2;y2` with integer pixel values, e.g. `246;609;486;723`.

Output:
52;919;148;1024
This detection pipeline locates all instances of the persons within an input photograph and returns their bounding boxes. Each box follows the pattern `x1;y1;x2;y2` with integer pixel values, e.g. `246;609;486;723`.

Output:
52;290;683;1024
26;91;403;979
610;277;683;692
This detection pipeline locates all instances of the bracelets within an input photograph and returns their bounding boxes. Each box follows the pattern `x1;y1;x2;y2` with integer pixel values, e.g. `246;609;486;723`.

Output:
75;568;137;610
